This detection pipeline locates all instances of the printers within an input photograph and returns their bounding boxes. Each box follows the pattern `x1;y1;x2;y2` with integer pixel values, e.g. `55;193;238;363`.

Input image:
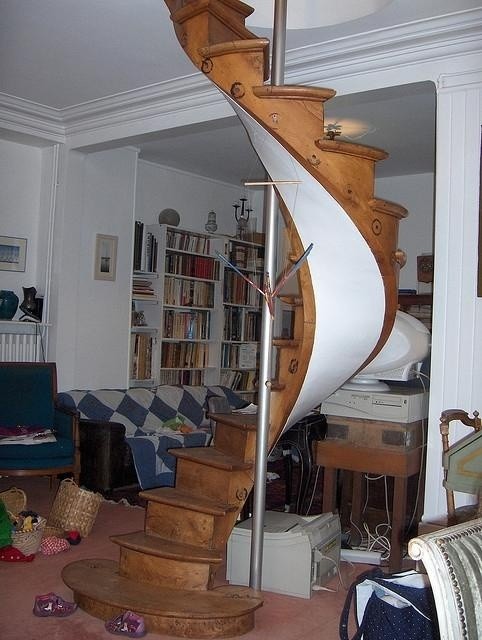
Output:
225;510;341;599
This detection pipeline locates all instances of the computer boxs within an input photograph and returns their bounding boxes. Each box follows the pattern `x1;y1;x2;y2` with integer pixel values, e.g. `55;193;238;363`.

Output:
320;385;429;424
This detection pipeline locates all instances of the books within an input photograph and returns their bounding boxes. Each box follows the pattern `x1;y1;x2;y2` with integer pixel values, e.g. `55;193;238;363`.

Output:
130;219;267;410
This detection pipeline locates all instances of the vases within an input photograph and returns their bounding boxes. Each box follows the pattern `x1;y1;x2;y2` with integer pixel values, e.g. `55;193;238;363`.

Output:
0;289;20;320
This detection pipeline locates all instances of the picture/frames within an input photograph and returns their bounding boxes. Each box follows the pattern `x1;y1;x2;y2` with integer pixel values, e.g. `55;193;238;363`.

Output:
93;233;119;282
0;235;28;273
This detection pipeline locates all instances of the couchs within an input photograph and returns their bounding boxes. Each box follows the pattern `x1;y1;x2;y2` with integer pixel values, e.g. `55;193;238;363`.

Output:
58;382;255;507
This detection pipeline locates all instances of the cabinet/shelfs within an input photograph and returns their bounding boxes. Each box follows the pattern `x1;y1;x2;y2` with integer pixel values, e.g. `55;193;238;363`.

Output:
132;220;265;406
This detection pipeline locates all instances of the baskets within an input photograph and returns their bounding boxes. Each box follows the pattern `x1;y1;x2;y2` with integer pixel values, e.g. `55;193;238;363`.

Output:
47;478;102;537
8;511;45;556
1;487;27;515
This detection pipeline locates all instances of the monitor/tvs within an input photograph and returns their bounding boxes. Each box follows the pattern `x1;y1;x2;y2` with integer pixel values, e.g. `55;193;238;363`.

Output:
341;309;431;392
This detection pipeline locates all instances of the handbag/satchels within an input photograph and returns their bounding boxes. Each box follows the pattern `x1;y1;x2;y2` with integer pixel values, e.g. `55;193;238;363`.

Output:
356;569;439;639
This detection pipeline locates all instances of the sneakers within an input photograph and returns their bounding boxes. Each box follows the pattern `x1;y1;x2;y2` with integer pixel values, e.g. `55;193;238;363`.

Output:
105;609;144;637
34;593;80;617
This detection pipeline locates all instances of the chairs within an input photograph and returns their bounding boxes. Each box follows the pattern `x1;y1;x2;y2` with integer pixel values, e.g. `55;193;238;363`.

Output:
407;516;481;639
0;359;81;486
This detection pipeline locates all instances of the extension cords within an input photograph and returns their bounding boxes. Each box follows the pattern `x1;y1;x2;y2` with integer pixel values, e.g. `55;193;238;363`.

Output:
341;549;382;566
